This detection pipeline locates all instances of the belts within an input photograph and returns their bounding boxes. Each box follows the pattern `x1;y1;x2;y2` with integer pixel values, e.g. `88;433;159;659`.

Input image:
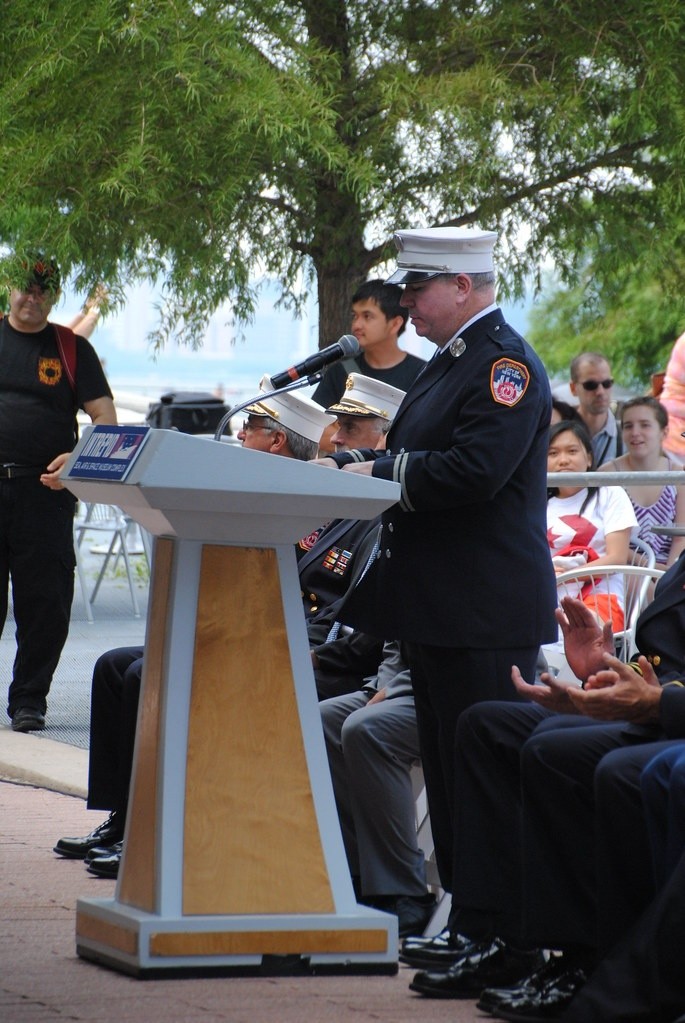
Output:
0;464;47;479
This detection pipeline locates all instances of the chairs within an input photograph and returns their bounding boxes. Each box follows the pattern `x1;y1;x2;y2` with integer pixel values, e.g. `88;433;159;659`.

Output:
70;502;154;621
528;540;664;687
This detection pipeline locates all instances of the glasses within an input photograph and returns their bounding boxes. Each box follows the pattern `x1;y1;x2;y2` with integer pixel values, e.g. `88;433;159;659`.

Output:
576;376;615;391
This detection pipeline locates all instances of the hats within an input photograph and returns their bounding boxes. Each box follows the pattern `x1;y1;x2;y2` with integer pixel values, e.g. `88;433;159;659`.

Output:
381;228;499;286
324;371;406;423
6;252;60;289
234;374;339;447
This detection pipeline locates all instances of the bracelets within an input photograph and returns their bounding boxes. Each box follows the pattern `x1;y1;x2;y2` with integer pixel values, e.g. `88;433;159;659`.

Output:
88;305;101;317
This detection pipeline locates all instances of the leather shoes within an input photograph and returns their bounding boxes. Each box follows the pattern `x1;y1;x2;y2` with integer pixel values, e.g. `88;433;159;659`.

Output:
86;851;122;880
408;936;547;998
475;963;564;1013
398;925;489;969
53;811;126;859
493;972;603;1023
11;701;46;731
383;891;438;935
84;840;126;864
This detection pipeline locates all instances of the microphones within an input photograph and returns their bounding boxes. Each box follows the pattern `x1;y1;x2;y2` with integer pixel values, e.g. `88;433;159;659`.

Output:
270;335;360;389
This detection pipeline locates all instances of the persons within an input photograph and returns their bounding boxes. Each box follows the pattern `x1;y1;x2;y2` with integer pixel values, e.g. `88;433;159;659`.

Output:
298;226;557;968
50;281;435;937
0;250;119;732
407;552;685;1023
546;332;685;638
